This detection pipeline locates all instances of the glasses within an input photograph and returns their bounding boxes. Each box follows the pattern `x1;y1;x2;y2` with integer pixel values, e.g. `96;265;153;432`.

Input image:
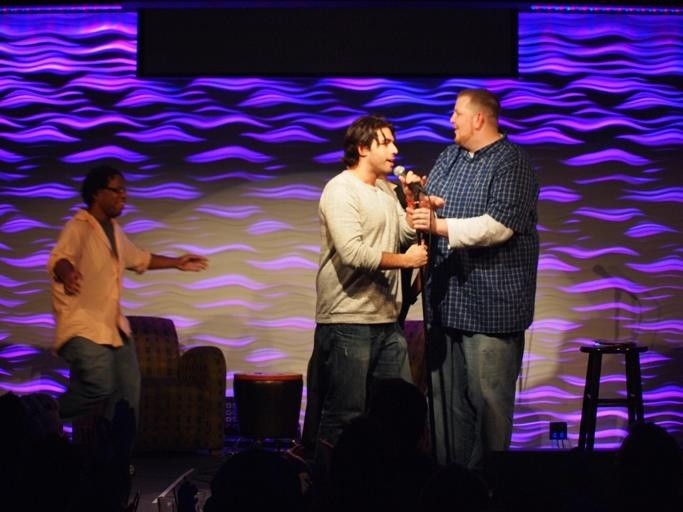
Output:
219;435;316;478
100;187;125;193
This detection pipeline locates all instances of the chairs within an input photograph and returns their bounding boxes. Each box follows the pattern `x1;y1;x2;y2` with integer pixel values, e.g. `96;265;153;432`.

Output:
118;316;227;462
305;320;428;430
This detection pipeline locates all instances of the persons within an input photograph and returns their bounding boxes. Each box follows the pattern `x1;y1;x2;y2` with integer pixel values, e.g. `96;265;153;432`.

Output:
47;164;210;422
406;88;539;485
0;377;683;512
300;113;429;511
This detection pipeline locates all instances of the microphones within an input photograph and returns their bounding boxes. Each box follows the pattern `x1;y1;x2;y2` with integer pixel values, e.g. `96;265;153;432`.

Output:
593;266;638;302
392;166;428;196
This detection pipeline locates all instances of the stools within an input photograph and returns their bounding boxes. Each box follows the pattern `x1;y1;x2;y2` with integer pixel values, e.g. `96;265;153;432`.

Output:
579;340;649;451
